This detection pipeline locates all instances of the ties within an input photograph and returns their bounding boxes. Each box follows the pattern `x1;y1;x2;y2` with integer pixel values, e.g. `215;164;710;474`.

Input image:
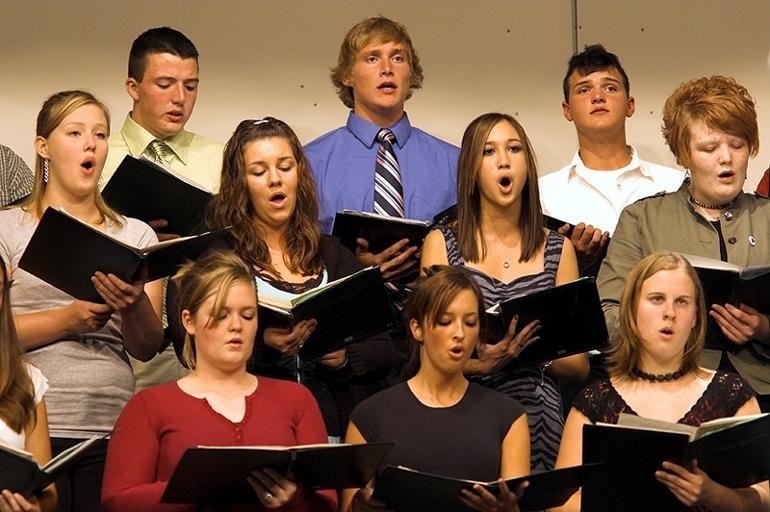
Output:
147;137;172;170
372;127;407;222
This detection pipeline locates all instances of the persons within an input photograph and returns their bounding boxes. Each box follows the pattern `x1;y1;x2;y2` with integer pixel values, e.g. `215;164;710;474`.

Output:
597;73;769;413
0;90;166;512
98;245;338;510
420;113;592;477
301;13;464;386
538;43;692;260
0;254;62;510
98;27;230;397
335;265;532;511
186;116;394;445
550;248;770;510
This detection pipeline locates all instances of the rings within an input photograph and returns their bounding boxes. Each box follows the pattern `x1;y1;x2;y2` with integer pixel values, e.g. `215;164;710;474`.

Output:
260;490;273;502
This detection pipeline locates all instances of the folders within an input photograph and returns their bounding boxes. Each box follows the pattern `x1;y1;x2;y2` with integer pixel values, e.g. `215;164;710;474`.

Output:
373;464;581;512
3;433;107;502
334;209;458;263
101;156;229;236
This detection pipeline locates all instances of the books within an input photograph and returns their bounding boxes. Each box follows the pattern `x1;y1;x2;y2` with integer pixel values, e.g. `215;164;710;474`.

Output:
542;213;612;278
369;453;584;511
0;423;107;502
102;151;215;238
331;200;457;282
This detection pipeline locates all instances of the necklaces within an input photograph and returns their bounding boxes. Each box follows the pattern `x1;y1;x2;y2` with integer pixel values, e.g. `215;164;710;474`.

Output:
688;192;741;210
628;361;692;384
481;210;525;271
